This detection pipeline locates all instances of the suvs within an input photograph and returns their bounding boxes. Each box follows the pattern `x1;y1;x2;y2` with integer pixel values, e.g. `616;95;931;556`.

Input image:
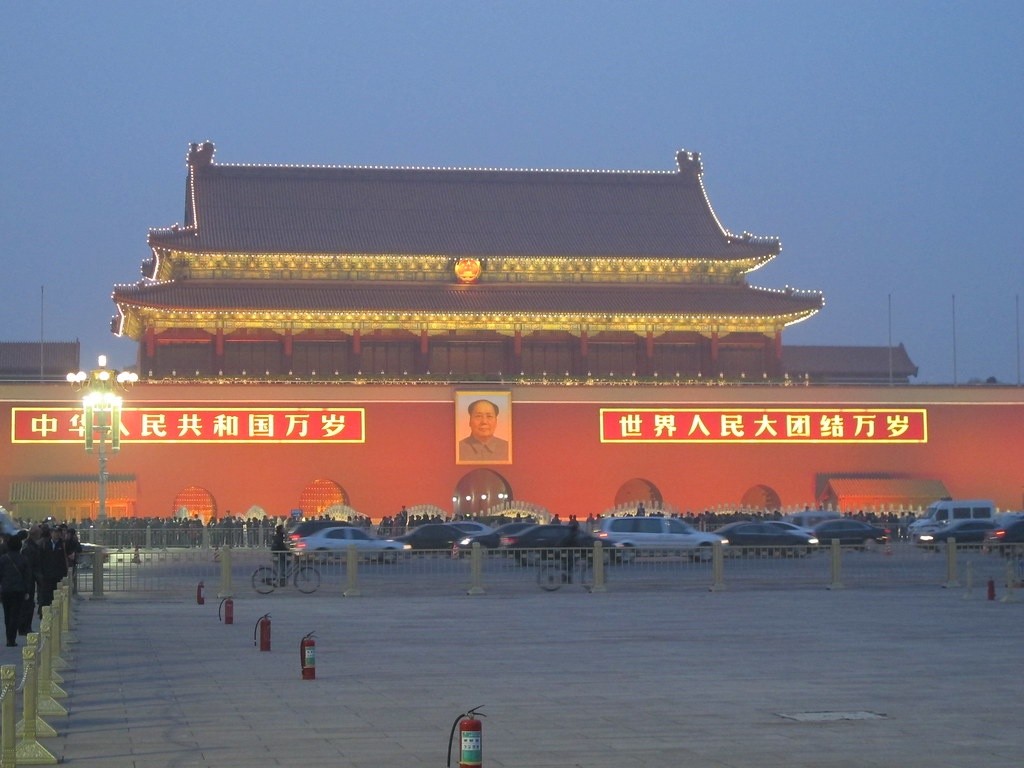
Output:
598;516;730;562
284;521;356;553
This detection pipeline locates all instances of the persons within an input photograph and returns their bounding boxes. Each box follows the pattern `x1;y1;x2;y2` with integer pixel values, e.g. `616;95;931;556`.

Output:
0;503;918;585
459;399;509;461
0;517;80;648
270;525;296;580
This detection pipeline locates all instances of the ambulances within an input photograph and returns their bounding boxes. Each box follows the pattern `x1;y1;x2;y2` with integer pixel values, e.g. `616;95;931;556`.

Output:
908;497;994;541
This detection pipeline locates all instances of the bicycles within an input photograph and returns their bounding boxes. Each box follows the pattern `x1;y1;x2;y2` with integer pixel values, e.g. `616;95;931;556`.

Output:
252;554;322;596
536;557;608;591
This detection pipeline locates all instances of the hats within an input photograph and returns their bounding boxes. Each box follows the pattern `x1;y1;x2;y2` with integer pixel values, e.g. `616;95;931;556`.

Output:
53;524;77;533
30;526;43;538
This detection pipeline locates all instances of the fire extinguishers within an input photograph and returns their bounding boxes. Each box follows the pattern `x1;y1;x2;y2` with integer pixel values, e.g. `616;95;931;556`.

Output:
300;630;317;679
218;595;234;624
446;704;487;768
254;612;272;652
196;580;204;605
987;580;995;601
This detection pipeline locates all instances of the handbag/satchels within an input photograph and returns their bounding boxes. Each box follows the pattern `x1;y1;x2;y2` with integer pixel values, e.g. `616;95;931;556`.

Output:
19;583;36;604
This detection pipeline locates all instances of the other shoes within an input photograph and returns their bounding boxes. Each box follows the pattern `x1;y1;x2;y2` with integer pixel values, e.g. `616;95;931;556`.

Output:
19;630;34;635
7;642;18;647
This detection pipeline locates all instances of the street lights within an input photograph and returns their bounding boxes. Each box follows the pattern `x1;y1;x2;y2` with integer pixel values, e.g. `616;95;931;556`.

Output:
66;354;140;548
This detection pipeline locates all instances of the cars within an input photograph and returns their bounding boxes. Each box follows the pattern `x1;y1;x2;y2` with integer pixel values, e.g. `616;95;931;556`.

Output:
393;524;472;555
919;520;996;548
983;520;1024;544
809;519;892;545
446;520;620;565
713;521;820;555
290;525;412;565
